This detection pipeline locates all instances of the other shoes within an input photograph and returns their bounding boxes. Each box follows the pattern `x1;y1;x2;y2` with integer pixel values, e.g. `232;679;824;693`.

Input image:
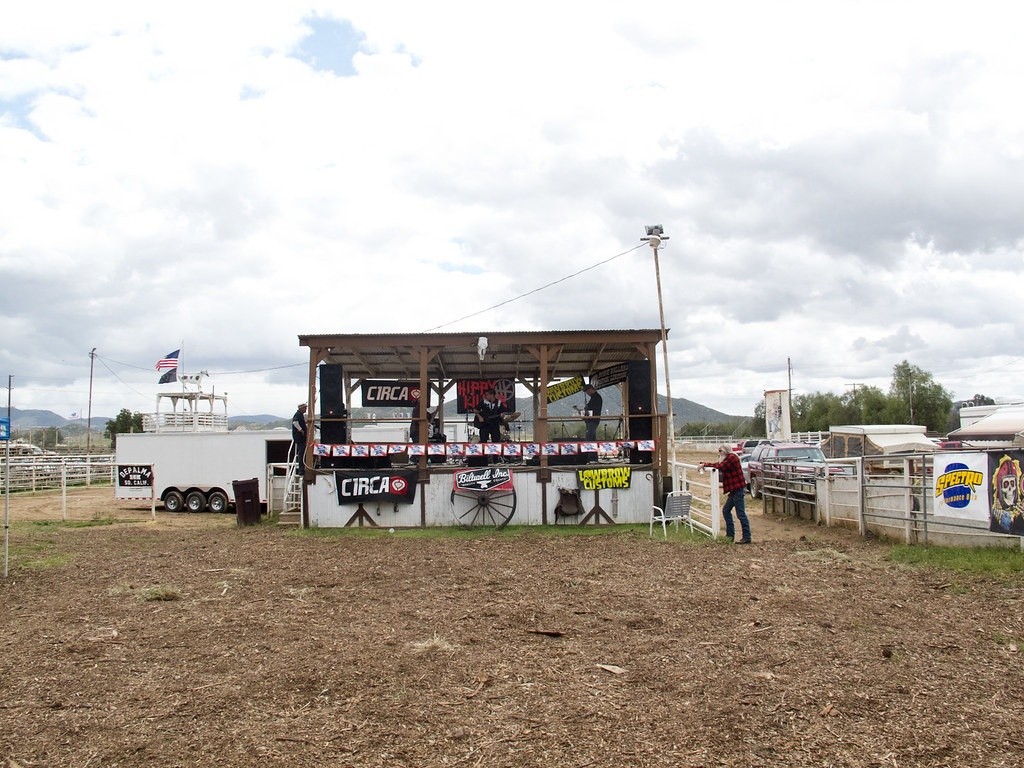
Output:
726;535;734;541
299;471;305;475
735;538;751;544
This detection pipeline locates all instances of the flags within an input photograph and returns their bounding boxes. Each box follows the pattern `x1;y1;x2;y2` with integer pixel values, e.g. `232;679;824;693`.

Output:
158;368;177;384
155;350;180;371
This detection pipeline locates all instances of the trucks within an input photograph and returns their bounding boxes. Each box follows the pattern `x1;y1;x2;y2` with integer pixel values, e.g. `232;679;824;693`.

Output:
828;424;943;473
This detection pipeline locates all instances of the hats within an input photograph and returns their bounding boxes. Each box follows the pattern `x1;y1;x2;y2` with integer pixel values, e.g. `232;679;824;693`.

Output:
583;385;593;392
298;404;308;408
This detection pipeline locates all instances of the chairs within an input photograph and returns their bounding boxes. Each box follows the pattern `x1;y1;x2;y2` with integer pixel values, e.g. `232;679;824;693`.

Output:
650;489;694;537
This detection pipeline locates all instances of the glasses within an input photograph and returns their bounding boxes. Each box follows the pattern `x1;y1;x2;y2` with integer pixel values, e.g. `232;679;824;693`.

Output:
719;450;722;452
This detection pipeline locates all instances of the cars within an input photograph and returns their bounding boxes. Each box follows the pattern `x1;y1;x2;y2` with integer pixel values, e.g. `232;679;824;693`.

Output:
866;437;963;478
718;436;833;494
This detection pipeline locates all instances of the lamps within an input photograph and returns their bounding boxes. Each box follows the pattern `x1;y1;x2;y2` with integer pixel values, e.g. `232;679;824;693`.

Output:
477;337;489;361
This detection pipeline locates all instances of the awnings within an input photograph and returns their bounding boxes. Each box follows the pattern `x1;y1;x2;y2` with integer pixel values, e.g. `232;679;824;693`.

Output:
866;434;942;454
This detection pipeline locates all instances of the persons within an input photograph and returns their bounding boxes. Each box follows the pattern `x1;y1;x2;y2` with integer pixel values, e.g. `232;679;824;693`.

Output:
697;444;752;544
583;384;602;440
292;403;308;475
410;398;439;443
474;390;506;442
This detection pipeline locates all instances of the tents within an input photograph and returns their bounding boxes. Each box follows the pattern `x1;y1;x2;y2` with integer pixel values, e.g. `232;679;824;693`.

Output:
946;408;1024;441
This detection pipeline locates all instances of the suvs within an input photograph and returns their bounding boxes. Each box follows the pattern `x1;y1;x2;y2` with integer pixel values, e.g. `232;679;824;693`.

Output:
747;442;846;502
